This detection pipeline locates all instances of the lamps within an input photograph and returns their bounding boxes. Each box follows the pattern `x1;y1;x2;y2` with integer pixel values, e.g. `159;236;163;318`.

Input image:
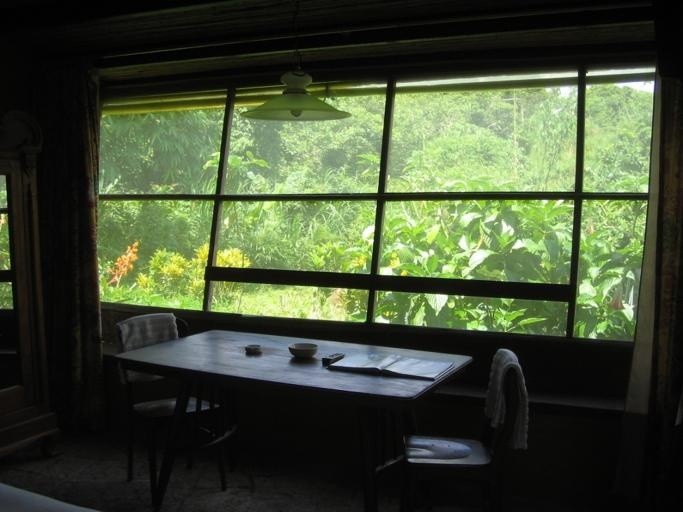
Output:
112;312;221;481
402;348;519;511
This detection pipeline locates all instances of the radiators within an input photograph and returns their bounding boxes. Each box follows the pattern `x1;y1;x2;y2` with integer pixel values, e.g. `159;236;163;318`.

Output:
321;352;345;363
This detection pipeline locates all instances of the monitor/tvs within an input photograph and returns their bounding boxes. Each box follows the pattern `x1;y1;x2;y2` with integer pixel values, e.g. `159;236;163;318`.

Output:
327;348;455;380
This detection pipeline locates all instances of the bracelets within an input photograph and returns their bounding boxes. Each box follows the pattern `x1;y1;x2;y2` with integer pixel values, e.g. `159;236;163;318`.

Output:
244;344;261;354
287;343;318;358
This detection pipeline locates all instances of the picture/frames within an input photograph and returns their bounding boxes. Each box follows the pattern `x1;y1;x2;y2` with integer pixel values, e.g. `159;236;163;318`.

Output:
114;328;473;512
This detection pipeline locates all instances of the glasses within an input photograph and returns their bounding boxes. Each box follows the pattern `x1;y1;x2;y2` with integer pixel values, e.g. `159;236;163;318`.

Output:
240;70;350;121
